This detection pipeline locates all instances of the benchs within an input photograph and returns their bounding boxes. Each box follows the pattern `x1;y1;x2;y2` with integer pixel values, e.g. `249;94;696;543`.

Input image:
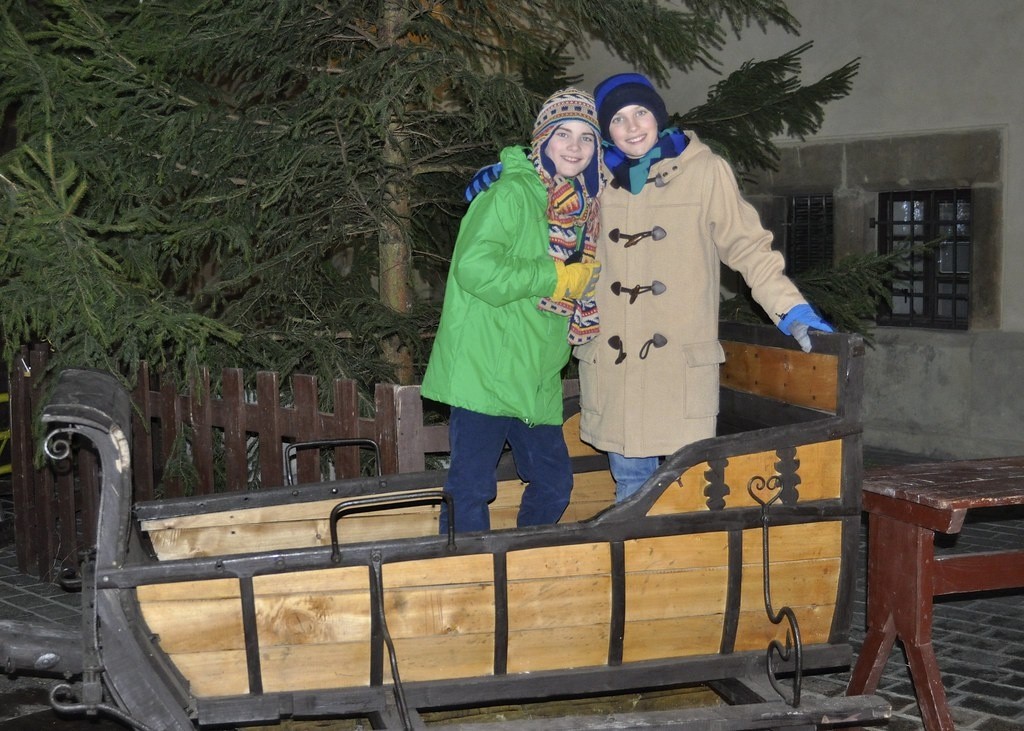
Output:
501;321;864;522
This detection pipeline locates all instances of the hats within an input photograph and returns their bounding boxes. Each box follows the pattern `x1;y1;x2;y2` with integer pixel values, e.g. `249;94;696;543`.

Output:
593;72;671;144
531;87;608;241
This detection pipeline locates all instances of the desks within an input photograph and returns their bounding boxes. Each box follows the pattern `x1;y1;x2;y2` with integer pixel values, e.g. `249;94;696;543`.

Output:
832;456;1024;731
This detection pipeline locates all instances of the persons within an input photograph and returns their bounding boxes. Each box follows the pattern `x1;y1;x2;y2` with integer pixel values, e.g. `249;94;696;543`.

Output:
465;72;837;502
420;86;607;535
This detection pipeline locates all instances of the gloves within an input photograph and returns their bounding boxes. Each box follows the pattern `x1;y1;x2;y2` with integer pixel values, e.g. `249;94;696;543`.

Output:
465;160;503;201
550;258;602;308
777;304;834;353
